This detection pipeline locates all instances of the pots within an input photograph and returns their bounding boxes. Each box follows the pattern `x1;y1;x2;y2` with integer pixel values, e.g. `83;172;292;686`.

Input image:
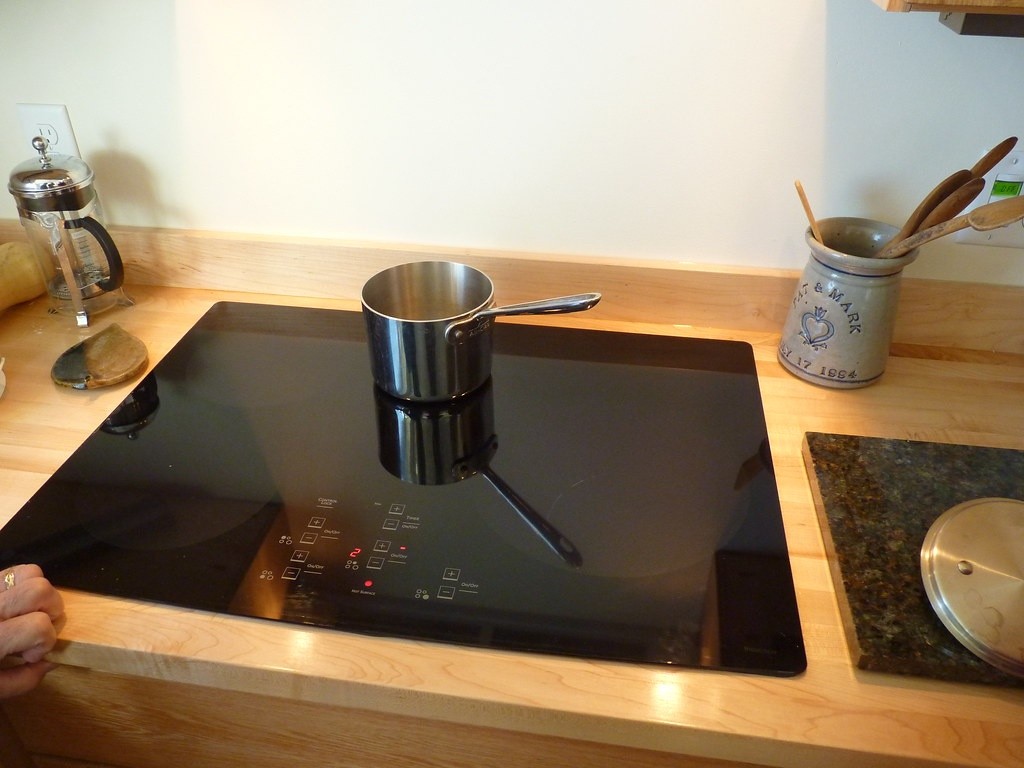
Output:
362;260;605;402
372;382;586;570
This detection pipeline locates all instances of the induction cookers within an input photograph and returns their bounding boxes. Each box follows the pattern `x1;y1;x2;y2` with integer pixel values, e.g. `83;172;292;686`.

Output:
0;301;808;678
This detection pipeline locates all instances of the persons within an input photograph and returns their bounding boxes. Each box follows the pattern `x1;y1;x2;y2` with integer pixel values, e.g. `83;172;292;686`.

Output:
0;564;64;697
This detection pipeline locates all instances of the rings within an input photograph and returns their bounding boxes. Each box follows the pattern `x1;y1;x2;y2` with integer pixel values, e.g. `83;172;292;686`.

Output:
5;570;15;590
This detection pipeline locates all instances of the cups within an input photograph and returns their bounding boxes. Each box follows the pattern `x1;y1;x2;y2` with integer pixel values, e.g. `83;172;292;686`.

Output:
7;138;136;327
777;217;921;389
